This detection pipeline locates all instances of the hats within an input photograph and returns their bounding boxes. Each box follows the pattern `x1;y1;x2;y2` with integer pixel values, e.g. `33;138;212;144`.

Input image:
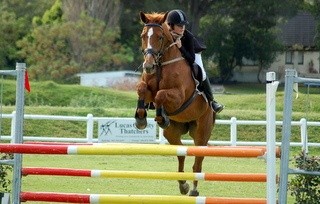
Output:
167;9;190;26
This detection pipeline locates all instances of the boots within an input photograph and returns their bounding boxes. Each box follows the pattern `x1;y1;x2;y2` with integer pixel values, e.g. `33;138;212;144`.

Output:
201;78;225;113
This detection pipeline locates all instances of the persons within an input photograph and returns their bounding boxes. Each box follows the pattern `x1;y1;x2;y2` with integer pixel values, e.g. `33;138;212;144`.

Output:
167;9;224;113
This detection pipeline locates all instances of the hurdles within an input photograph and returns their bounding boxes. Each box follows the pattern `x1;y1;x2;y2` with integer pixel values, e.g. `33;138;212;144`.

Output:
280;68;320;204
0;62;277;204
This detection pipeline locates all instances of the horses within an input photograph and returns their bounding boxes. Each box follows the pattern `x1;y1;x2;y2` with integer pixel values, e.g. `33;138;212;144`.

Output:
134;10;216;196
